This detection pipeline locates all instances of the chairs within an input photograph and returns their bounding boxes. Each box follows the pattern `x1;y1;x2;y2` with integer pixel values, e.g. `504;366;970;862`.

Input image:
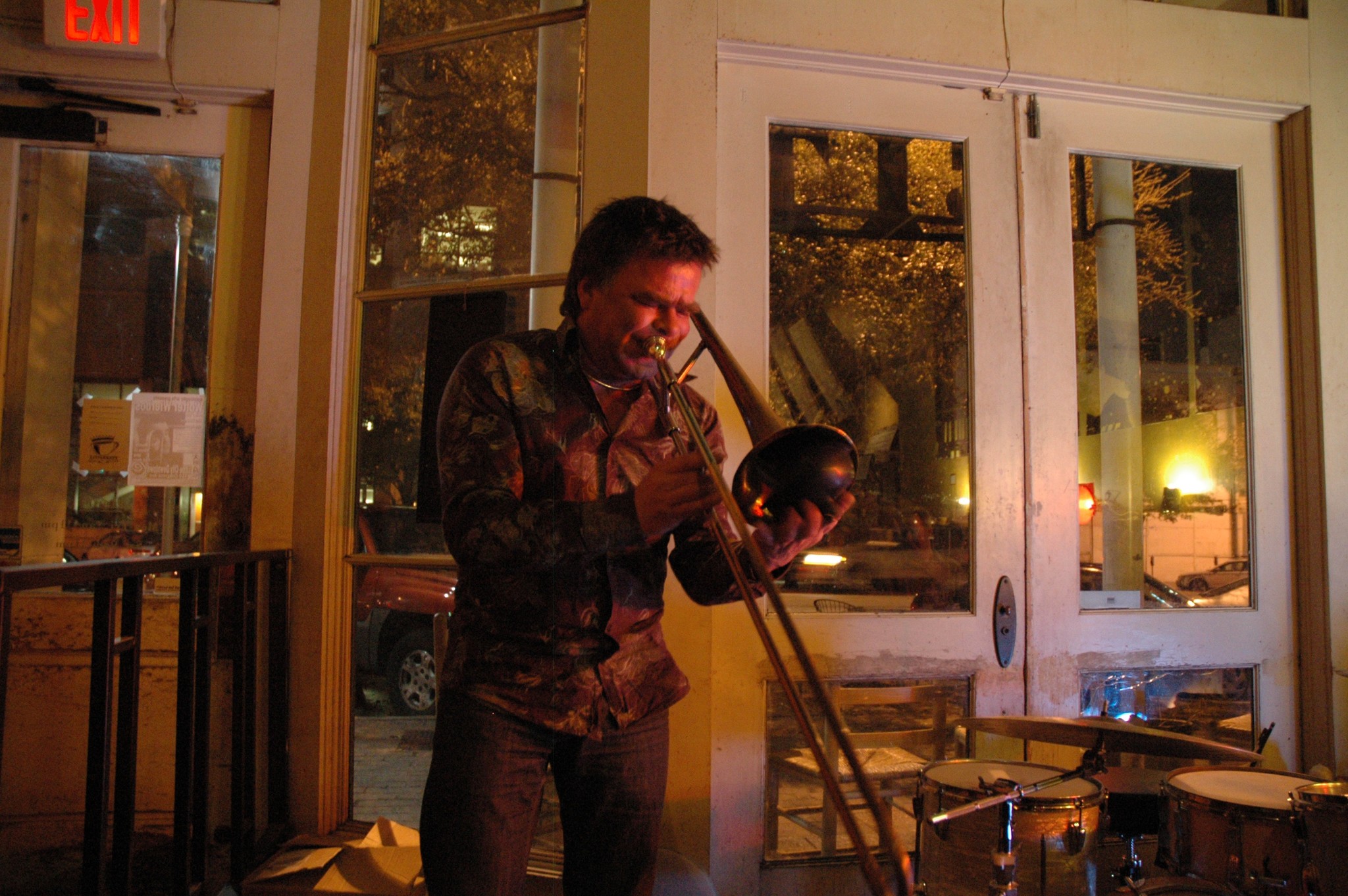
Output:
764;678;952;859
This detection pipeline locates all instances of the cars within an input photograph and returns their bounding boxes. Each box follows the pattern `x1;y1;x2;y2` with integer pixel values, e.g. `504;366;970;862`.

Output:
82;531;163;577
0;527;90;594
206;503;463;714
171;531;208;573
905;562;1249;700
789;538;952;593
1175;558;1250;595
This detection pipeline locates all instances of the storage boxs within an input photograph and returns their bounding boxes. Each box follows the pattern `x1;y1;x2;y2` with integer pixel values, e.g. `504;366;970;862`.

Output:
241;816;425;896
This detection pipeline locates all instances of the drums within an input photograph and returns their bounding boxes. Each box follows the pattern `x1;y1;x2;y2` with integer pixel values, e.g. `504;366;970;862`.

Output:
1094;832;1159;895
1295;778;1348;895
921;759;1105;896
1159;765;1339;895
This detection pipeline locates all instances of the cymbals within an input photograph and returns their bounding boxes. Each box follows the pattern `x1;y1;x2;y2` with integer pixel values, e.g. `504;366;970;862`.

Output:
953;714;1266;762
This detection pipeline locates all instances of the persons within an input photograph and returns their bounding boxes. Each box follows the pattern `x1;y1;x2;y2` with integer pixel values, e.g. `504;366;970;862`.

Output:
419;196;856;896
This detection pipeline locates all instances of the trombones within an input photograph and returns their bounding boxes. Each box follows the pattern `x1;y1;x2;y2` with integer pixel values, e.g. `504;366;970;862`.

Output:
641;302;914;896
